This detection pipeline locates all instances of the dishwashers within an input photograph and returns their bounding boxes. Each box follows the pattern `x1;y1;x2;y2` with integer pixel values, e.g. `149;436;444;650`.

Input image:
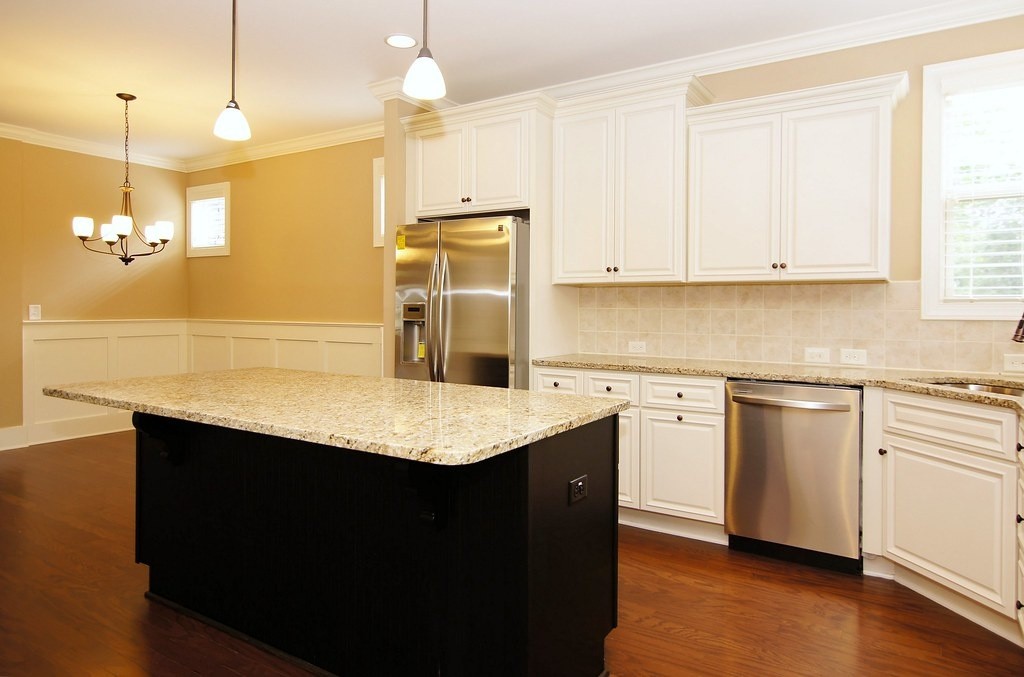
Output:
721;376;863;562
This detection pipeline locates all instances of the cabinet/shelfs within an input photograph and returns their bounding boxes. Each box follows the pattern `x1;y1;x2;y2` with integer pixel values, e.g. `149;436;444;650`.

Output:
686;70;911;283
531;353;732;547
881;371;1024;649
550;72;717;286
398;90;559;222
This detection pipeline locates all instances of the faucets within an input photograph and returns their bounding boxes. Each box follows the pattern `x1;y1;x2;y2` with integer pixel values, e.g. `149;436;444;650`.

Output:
1011;311;1024;343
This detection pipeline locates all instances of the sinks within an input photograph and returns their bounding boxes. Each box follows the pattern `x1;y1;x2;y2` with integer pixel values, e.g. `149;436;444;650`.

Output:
899;376;1024;398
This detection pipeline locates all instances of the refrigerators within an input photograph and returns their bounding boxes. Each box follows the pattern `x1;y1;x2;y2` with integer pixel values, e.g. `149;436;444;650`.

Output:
390;214;528;388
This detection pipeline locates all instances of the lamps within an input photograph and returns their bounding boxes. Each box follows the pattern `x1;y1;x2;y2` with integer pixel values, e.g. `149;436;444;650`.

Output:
72;92;175;267
211;0;252;142
402;0;447;100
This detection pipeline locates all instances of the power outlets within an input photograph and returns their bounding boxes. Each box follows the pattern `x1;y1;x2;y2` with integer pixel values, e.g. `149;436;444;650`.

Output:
839;349;868;364
804;347;830;363
629;342;646;354
28;304;42;320
1004;354;1024;373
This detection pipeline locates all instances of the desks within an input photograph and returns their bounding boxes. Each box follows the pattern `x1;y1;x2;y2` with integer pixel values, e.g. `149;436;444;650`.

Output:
40;370;632;677
